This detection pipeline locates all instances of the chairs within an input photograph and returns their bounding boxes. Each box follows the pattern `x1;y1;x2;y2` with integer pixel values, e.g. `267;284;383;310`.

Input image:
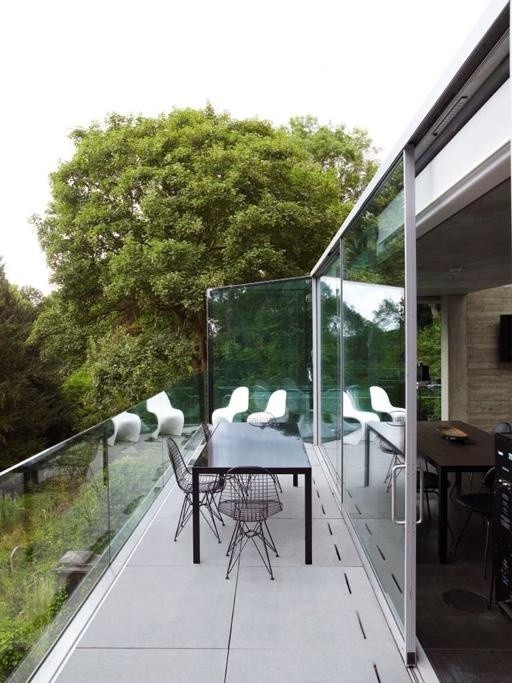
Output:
107;409;142;448
448;465;498;574
167;436;225;544
380;441;404;492
211;386;251;425
246;389;289;425
369;384;406;425
491;421;510;434
198;419;249;504
341;390;381;444
43;433;105;527
253;378;362;437
245;410;284;494
144;389;185;441
416;469;449;524
216;465;286;579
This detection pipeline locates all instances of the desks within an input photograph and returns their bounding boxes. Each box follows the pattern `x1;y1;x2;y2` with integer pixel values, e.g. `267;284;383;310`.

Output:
413;415;504;560
192;422;315;564
363;423;404;489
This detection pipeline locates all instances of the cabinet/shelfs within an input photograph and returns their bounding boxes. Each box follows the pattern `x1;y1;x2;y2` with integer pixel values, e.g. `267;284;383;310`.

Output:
493;434;511;620
